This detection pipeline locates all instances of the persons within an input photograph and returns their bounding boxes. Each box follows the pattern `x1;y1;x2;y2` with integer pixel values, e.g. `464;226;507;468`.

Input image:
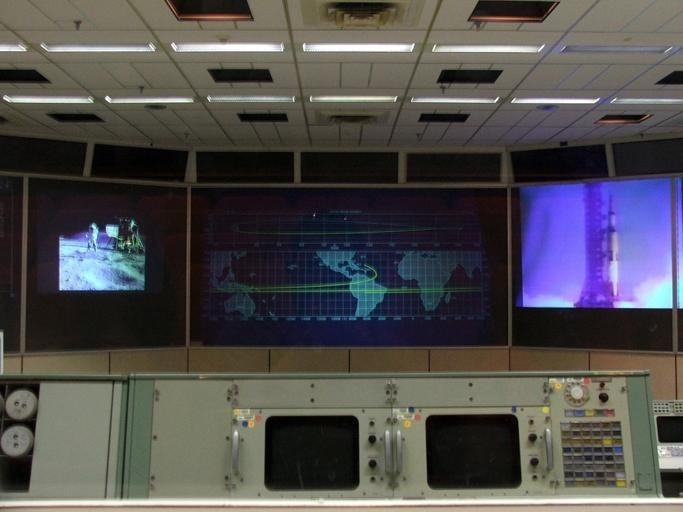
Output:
85;223;99;252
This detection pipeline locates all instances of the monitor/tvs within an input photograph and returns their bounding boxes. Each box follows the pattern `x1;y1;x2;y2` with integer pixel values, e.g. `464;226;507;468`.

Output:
509;173;676;355
23;174;189;357
672;173;683;355
0;172;24;357
188;183;510;348
